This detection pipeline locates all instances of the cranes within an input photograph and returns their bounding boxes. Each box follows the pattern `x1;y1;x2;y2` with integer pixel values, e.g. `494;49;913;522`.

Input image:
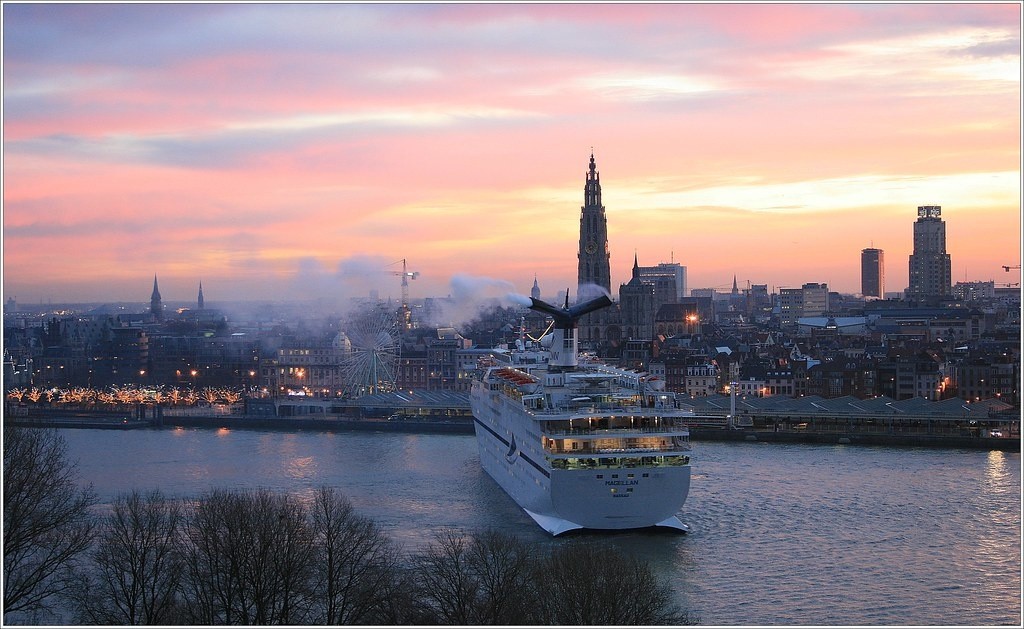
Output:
1002;264;1021;272
353;258;420;326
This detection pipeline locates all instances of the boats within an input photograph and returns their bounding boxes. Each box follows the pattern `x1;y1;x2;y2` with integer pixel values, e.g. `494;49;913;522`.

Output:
468;292;696;537
